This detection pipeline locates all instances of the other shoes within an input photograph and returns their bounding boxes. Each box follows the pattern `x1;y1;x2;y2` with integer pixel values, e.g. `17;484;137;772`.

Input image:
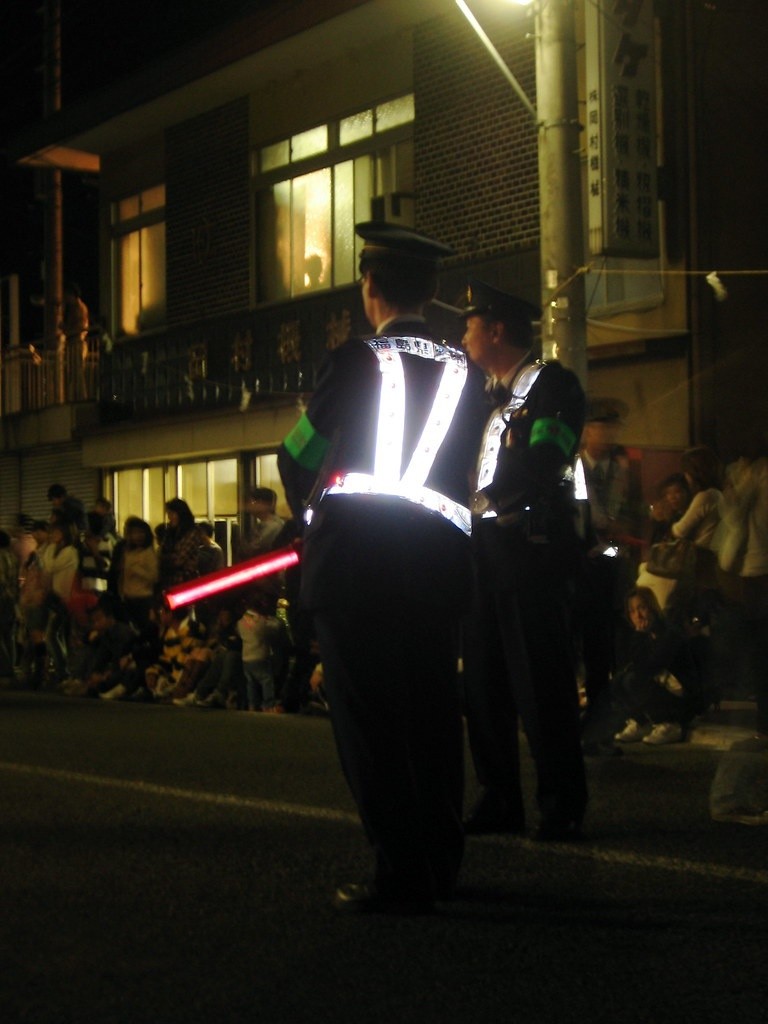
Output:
532;799;581;838
461;811;526;834
95;680;330;722
334;875;433;914
579;740;623;759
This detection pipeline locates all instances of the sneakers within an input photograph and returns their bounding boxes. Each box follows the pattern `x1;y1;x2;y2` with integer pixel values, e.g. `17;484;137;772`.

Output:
612;717;651;743
641;720;682;744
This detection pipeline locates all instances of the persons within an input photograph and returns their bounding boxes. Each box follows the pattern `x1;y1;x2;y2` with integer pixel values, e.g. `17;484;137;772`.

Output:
0;284;766;838
278;223;494;913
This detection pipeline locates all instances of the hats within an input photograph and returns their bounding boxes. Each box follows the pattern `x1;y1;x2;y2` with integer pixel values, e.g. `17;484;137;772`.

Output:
458;279;543;330
355;220;458;267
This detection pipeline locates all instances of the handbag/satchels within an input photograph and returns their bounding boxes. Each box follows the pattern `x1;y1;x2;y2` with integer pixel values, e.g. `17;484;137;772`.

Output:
645;525;696;582
